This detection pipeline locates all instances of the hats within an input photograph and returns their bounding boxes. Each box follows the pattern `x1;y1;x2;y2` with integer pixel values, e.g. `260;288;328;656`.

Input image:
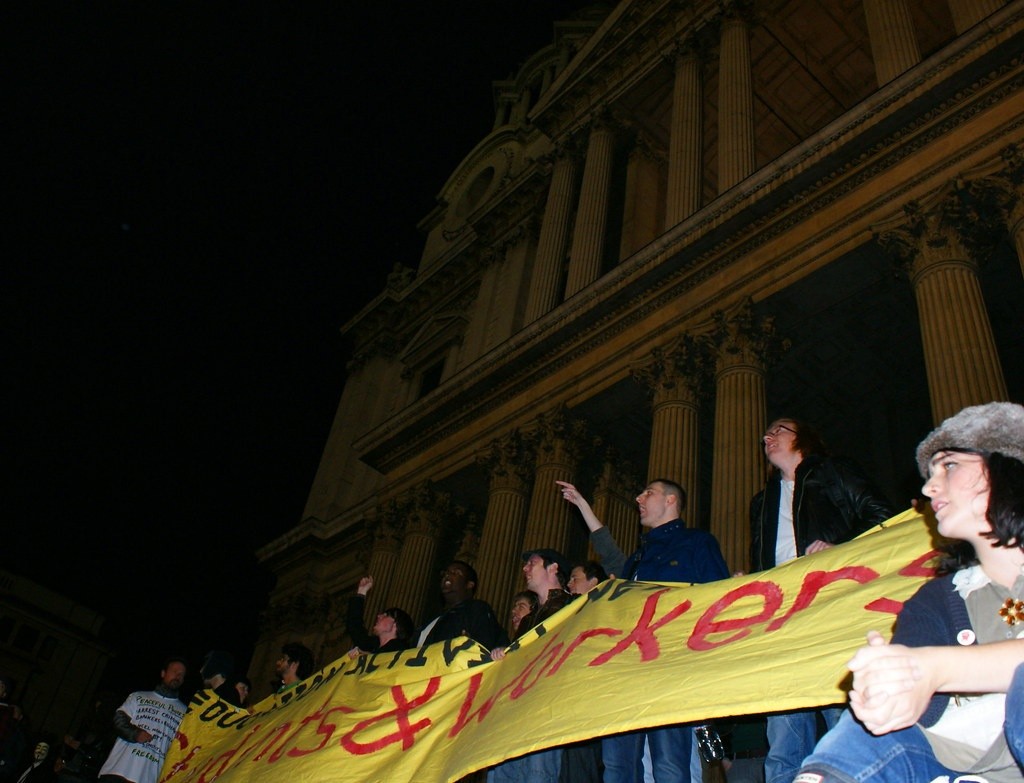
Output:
914;401;1023;481
523;548;574;574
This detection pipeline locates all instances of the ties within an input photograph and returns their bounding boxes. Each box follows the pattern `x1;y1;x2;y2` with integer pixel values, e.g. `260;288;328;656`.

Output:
18;764;34;782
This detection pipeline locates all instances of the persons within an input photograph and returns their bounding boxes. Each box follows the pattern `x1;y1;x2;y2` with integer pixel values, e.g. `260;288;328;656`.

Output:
1;703;68;783
753;415;892;783
198;657;233;707
492;588;559;783
601;478;735;783
556;478;712;783
562;557;602;598
410;560;512;783
230;676;252;709
273;638;318;690
793;395;1024;783
347;573;410;661
93;660;191;783
513;547;600;783
485;766;494;783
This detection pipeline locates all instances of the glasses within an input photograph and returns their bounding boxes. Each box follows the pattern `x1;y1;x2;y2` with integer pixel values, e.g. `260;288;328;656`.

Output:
760;425;797;444
438;568;467;582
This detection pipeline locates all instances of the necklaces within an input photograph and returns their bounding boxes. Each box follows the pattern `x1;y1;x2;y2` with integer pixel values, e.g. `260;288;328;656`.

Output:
968;547;1024;626
